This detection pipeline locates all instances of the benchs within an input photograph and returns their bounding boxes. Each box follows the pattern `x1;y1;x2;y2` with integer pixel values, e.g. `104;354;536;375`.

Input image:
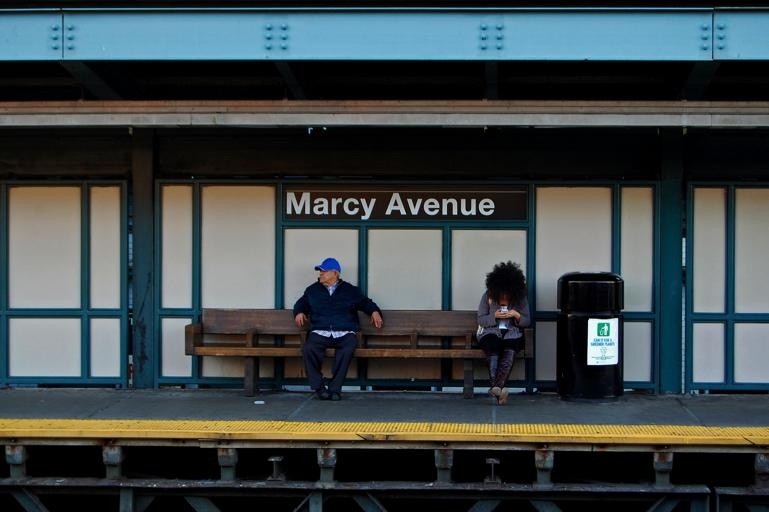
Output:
184;307;534;400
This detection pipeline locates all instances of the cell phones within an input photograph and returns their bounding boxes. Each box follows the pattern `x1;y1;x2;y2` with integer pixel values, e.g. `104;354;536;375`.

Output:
501;309;508;313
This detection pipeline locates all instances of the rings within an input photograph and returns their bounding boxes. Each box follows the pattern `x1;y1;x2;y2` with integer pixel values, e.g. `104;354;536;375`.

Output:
379;320;382;322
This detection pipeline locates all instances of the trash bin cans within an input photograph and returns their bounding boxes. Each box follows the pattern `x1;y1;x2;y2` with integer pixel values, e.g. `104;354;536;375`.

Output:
555;271;624;403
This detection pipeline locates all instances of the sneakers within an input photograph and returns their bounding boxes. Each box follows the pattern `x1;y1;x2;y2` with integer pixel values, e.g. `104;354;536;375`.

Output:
316;387;329;401
329;392;341;402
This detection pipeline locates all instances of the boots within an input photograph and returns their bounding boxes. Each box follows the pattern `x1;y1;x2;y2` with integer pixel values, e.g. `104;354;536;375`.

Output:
488;349;516;407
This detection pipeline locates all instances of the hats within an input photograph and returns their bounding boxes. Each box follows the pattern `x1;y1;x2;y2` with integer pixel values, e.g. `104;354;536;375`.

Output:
314;257;341;274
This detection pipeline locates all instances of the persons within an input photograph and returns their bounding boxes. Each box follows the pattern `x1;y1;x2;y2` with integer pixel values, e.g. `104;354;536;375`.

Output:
474;259;530;407
291;257;384;401
